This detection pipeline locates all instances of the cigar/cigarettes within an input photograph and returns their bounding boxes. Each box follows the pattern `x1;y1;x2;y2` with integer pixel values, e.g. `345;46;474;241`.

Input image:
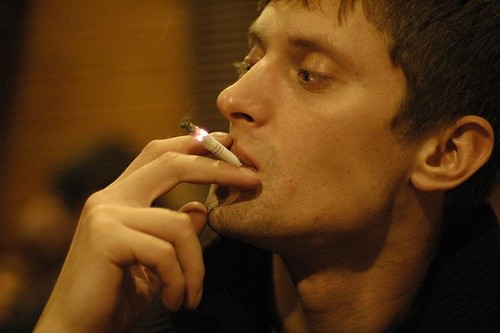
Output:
179;121;243;167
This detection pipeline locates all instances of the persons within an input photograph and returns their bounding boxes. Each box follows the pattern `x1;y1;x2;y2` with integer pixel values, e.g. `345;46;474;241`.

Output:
31;0;500;333
